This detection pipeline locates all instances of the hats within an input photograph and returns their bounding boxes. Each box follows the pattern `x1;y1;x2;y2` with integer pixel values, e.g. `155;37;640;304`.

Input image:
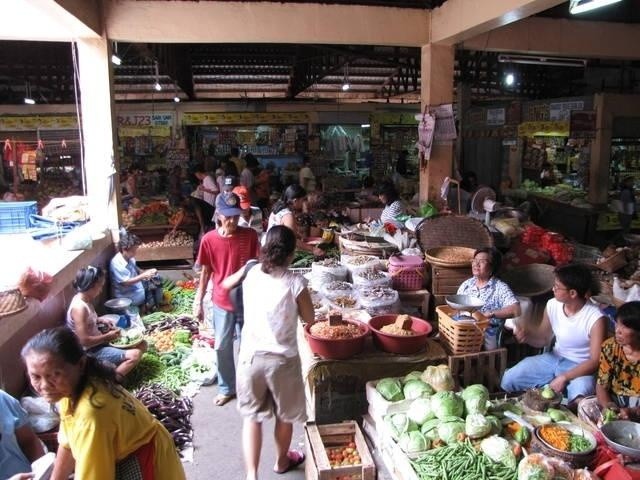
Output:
215;174;252;217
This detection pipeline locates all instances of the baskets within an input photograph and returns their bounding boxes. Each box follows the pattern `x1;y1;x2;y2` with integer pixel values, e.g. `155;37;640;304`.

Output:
531;425;596;470
388;255;426;291
435;305;490;355
594;249;628;274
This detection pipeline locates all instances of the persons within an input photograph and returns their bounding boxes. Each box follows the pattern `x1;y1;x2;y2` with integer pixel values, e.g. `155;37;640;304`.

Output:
66;263;148;386
120;142;419;255
459;140;639;272
107;231;173;315
222;223;313;480
3;165;74;201
578;299;640;427
500;262;608;409
191;189;264;408
455;247;522;355
22;322;187;480
0;387;54;480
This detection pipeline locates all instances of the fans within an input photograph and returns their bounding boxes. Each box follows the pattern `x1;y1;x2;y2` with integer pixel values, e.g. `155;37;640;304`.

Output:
471;186;500;230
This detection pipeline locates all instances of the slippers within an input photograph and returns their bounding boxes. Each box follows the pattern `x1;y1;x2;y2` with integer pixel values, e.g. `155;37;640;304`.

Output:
273;449;305;475
213;393;236;406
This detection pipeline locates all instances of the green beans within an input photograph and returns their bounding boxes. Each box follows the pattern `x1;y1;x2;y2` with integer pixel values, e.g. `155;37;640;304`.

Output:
408;435;518;480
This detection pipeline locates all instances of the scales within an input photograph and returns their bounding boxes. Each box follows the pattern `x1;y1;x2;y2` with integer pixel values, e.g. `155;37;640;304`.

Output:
445;294;485;323
600;419;640;480
103;296;140;316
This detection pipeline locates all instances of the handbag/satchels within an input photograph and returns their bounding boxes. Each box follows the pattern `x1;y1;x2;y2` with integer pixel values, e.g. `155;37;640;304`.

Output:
228;262;260;314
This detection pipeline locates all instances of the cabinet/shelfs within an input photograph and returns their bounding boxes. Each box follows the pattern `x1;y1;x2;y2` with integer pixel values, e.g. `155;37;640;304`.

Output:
429;265;474;321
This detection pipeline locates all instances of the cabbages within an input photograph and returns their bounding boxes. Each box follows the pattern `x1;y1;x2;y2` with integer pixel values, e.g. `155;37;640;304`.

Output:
375;370;503;451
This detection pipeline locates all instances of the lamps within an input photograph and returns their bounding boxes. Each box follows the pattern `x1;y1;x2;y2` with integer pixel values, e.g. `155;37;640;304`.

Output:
109;41;184;105
22;77;35;105
342;61;351;92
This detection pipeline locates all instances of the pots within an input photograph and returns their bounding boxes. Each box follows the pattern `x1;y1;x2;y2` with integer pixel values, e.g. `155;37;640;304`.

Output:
503;410;597;464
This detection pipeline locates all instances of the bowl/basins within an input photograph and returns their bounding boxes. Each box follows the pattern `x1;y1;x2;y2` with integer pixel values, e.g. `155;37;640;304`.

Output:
600;418;640;458
368;315;434;355
304;318;368;356
536;422;597;454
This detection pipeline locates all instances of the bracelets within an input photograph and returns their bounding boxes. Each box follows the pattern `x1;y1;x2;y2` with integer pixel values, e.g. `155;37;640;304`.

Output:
196;298;203;306
488;307;496;321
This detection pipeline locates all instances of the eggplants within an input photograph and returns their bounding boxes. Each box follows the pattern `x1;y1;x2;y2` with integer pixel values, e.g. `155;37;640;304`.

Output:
133;383;194;445
145;316;200;335
